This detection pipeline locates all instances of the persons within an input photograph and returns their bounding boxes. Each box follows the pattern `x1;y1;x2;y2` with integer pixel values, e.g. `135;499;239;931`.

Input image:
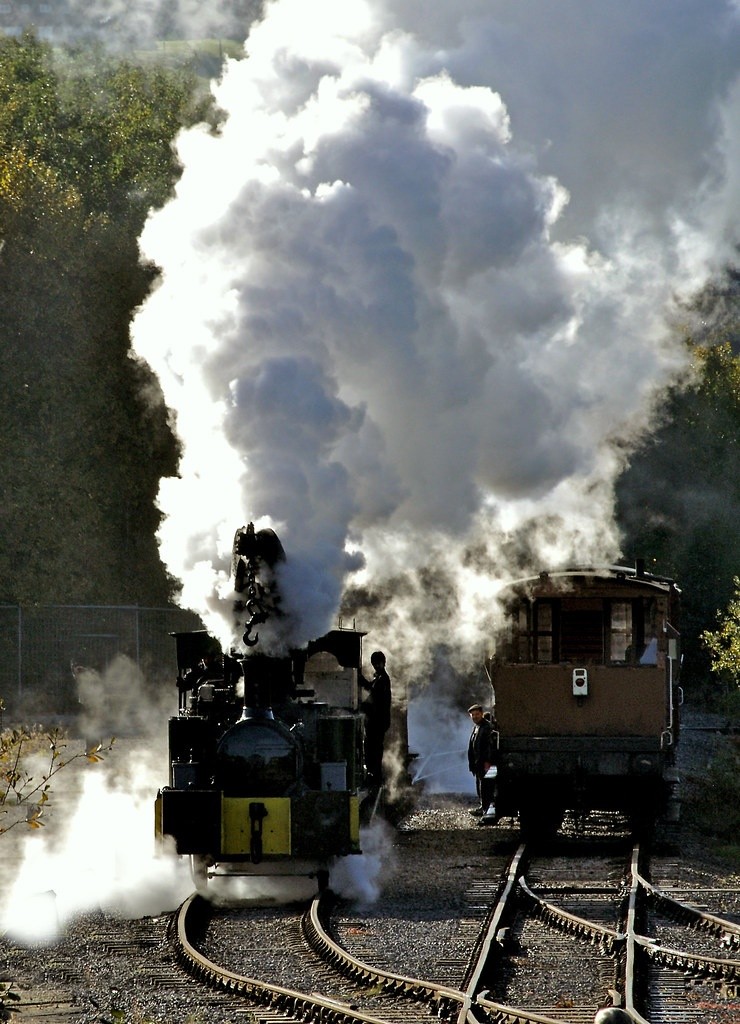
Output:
360;652;391;787
468;704;494;816
176;652;225;694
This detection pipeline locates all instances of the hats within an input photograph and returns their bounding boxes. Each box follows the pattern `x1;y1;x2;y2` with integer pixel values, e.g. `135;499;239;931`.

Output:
467;704;482;712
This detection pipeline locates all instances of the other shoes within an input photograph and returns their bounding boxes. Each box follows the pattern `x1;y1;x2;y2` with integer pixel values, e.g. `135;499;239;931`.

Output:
468;806;484;817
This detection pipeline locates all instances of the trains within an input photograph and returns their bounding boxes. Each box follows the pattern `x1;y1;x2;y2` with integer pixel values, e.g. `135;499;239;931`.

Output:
483;546;686;839
154;526;411;902
